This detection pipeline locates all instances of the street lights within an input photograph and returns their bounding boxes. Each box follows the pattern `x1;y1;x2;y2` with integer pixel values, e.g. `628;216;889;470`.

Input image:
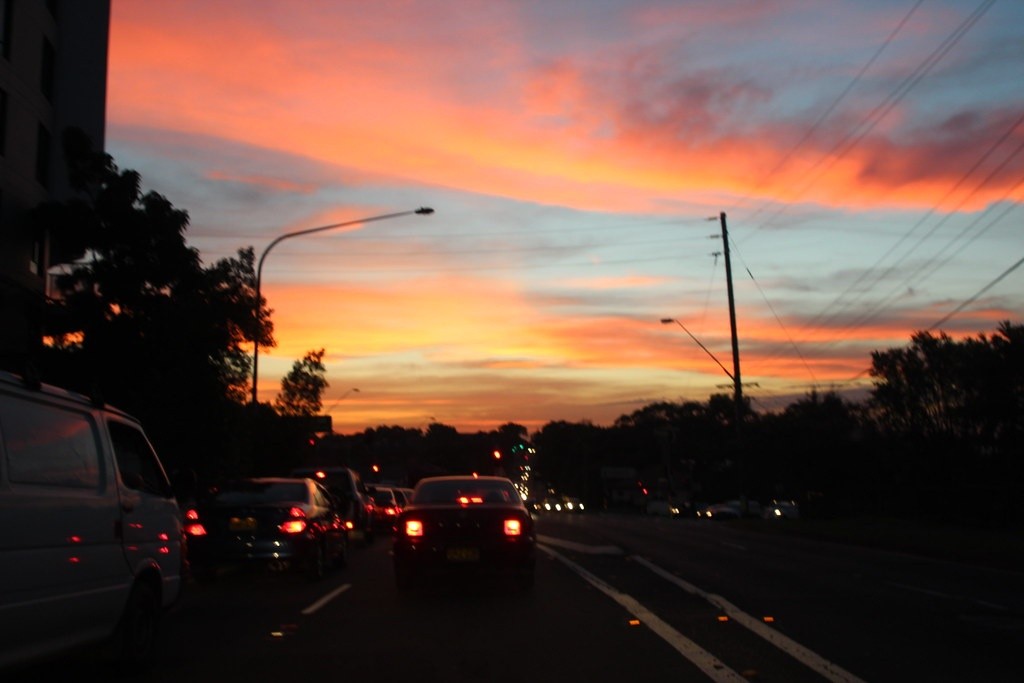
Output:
661;316;742;399
252;204;434;398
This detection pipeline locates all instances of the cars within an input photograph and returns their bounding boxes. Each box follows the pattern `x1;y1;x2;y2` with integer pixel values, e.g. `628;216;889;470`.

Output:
669;501;737;521
292;464;416;543
386;475;536;596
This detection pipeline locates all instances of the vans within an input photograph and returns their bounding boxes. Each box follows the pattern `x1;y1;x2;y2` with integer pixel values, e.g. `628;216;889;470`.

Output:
0;370;191;683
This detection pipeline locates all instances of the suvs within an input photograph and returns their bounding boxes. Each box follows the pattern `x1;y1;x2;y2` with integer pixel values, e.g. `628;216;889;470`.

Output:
184;475;355;584
